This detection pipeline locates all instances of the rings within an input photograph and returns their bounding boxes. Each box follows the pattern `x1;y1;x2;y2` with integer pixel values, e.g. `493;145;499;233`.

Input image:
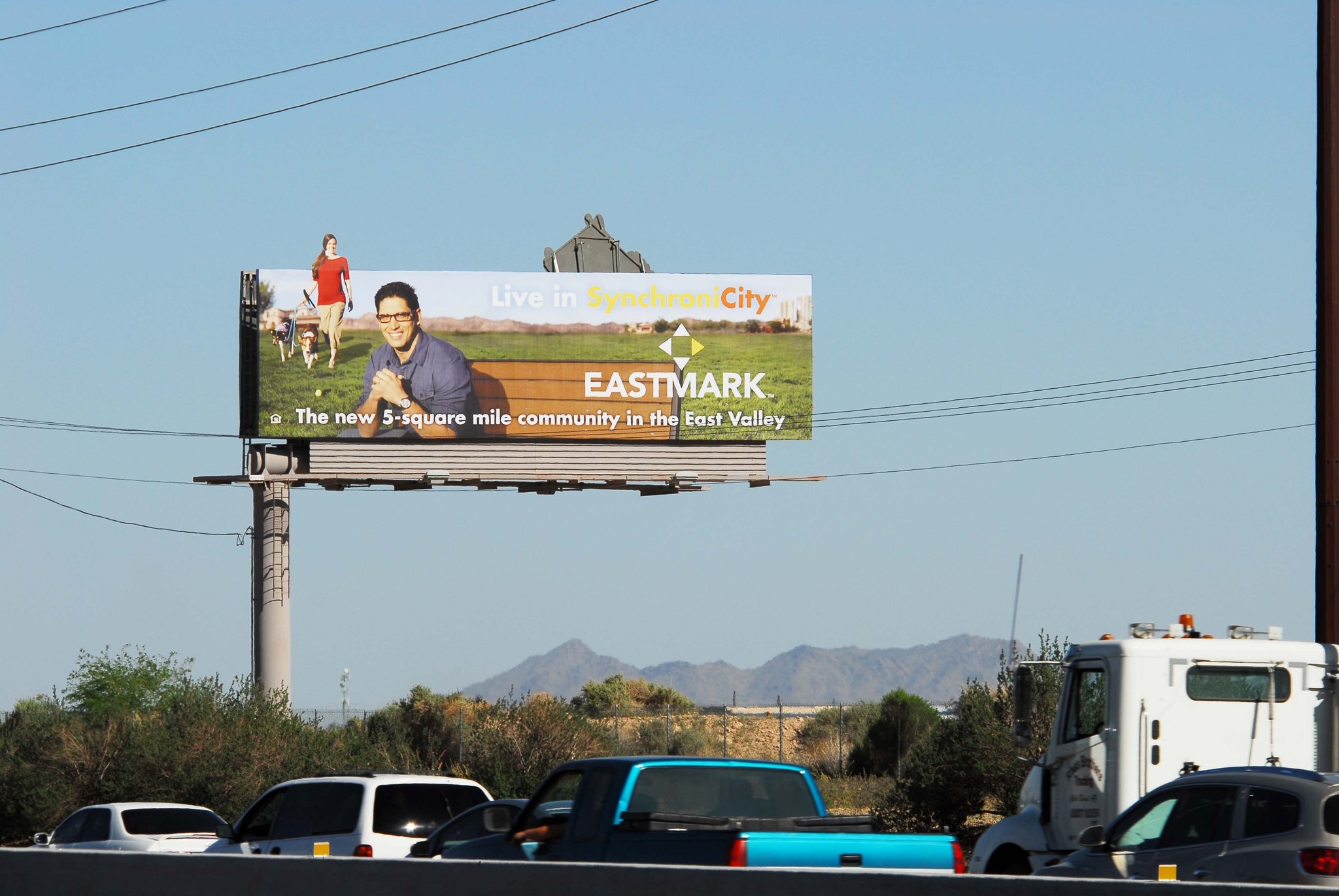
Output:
378;379;383;385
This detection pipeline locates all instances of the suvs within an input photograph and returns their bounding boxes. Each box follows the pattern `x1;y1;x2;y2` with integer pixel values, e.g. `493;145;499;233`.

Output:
204;770;495;858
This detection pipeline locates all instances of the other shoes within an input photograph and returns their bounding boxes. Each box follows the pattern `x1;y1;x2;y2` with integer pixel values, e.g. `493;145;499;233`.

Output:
328;361;336;369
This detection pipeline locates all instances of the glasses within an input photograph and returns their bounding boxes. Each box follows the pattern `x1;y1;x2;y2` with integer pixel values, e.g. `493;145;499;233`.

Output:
376;309;415;323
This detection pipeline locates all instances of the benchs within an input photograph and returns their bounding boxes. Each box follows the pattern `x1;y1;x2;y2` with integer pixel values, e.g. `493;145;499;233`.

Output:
395;360;683;440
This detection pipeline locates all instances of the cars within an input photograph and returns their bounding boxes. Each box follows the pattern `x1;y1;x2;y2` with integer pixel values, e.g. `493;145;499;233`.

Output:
1037;766;1339;888
405;798;574;860
25;802;229;853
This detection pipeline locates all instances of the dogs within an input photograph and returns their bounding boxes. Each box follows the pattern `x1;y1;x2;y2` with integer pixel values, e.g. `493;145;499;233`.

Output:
298;323;319;368
269;319;296;362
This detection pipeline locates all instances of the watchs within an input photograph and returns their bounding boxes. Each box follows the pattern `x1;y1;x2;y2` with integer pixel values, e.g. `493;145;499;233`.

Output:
398;395;413;411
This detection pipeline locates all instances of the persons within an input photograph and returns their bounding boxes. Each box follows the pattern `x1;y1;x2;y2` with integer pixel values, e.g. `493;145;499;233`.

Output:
332;281;485;438
296;233;353;369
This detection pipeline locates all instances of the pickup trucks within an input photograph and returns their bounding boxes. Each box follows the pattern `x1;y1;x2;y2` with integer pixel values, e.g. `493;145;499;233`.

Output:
440;755;965;875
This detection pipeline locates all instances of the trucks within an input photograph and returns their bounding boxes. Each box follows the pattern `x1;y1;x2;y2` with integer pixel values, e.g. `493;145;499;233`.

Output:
968;614;1339;876
